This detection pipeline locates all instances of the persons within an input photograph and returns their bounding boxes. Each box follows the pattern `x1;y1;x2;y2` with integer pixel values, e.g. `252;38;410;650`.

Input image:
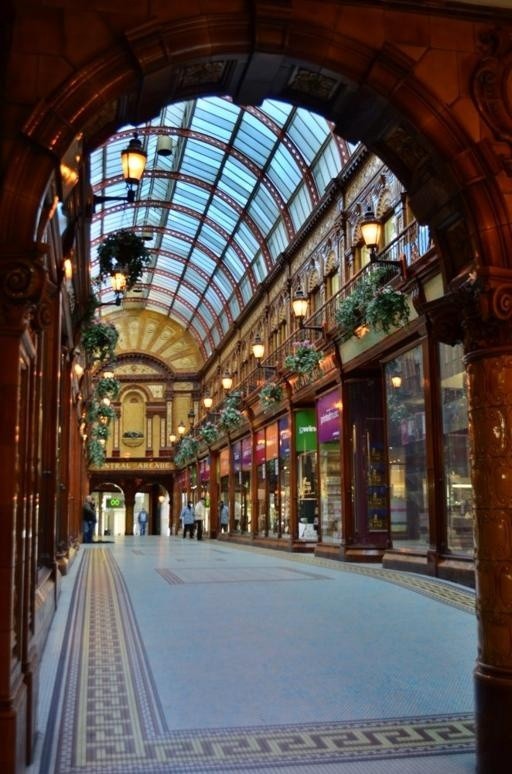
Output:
89;498;95;511
83;496;95;542
219;500;229;533
193;497;205;541
137;507;148;536
180;500;195;538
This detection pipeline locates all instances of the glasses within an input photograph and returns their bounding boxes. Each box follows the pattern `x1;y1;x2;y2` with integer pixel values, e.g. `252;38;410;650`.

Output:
156;106;172;157
88;126;149;205
250;332;279;377
292;286;325;341
165;363;241;452
359;202;409;282
89;263;129;310
